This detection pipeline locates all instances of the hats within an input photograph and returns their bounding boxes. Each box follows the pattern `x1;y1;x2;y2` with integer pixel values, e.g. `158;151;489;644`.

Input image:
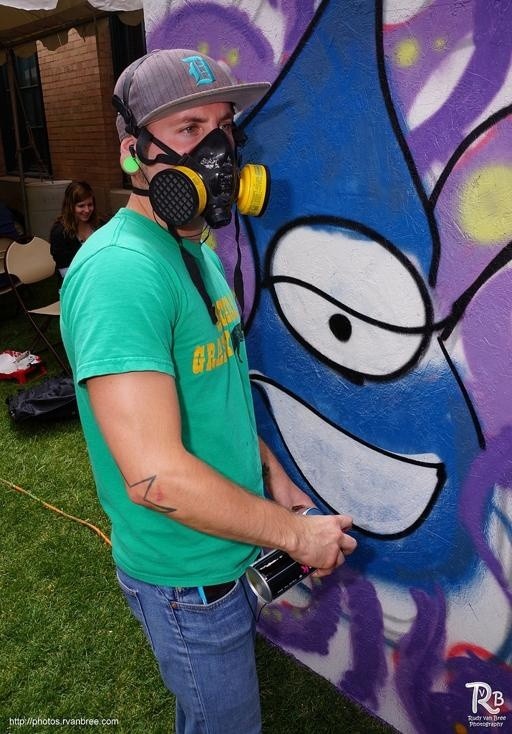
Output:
112;48;271;142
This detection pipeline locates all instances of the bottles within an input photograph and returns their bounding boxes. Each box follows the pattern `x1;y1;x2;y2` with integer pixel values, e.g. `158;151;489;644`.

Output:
244;548;319;607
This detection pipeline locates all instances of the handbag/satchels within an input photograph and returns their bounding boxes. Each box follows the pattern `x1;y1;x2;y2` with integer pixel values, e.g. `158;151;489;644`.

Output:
5;375;78;425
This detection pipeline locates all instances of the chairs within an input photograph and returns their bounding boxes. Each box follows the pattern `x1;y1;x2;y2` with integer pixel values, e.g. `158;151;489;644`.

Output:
5;234;72;385
